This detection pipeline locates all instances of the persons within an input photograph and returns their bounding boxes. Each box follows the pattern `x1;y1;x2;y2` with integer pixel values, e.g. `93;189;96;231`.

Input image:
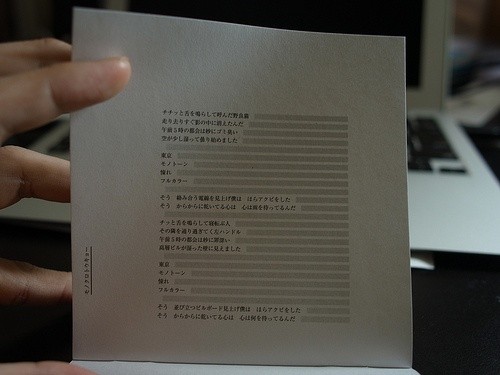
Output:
0;37;131;373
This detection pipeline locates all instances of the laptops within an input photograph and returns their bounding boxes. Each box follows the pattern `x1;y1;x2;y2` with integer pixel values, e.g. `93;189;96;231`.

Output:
0;0;500;257
